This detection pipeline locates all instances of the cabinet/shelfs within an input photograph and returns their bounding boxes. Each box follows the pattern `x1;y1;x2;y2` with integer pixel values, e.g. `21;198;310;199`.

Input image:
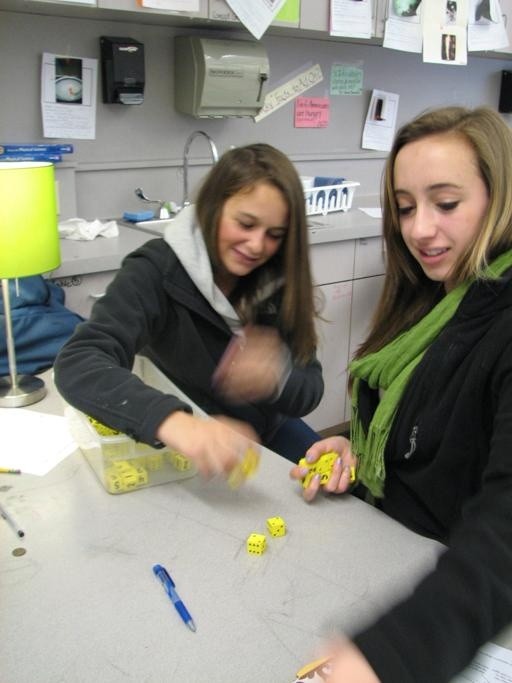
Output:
210;2;302;37
299;1;380;44
467;0;510;62
302;237;389;437
53;2;97;19
381;1;468;42
98;1;209;26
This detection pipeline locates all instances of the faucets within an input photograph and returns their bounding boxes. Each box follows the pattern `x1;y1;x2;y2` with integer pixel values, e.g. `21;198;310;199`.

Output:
183;130;219;199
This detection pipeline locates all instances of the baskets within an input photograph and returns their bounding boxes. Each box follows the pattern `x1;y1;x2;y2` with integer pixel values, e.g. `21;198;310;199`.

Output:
300;176;360;216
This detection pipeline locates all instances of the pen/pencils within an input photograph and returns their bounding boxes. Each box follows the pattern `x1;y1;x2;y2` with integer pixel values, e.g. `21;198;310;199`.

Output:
0;468;21;474
153;564;197;634
0;507;24;537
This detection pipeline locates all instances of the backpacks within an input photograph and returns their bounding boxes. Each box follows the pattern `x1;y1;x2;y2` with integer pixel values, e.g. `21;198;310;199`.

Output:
0;274;88;377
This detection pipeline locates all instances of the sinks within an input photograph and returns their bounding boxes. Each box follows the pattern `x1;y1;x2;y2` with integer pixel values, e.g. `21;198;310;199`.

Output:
137;219;174;237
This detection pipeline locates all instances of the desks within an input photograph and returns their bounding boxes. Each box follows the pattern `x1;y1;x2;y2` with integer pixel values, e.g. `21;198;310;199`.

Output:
56;275;126;319
0;340;512;683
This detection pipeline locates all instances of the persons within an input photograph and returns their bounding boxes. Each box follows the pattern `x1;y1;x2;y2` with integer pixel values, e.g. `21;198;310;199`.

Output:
53;142;370;501
289;104;511;682
